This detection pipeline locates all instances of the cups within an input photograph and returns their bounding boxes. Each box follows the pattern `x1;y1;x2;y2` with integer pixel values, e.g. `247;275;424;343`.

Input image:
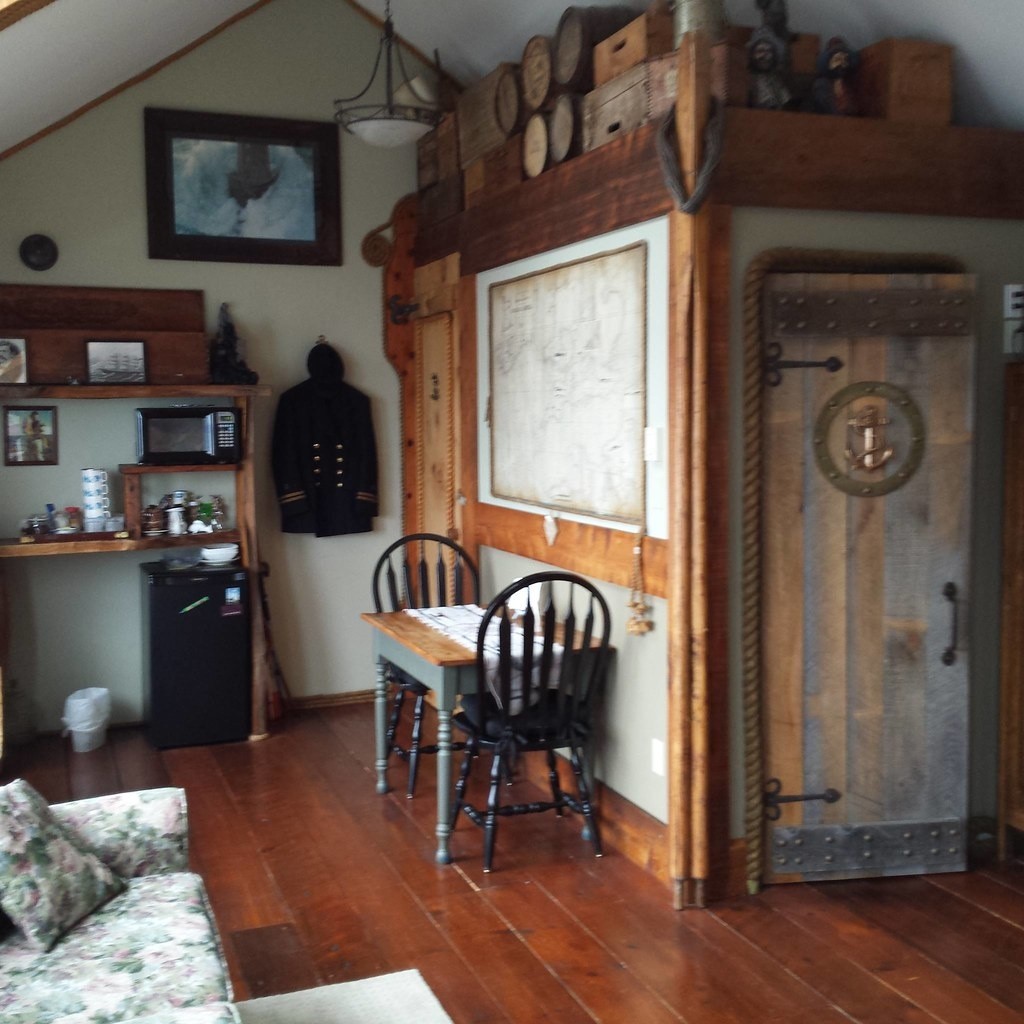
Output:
80;468;110;531
105;521;124;531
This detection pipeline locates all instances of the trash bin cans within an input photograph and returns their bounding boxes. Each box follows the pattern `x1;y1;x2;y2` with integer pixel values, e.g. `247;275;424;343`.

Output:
64;687;109;750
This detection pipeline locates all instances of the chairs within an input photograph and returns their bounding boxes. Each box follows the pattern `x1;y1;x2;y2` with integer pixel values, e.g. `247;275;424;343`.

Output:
444;571;611;872
372;534;513;798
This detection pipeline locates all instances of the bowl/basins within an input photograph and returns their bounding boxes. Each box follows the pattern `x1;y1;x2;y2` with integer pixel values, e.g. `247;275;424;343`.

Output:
201;542;239;560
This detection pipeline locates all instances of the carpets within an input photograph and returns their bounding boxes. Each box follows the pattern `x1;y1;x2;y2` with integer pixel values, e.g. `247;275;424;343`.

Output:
235;969;456;1024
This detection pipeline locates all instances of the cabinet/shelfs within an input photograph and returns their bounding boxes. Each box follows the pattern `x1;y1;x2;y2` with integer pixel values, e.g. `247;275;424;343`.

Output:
0;283;271;760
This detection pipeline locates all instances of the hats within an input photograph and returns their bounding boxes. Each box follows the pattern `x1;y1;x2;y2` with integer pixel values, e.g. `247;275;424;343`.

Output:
308;343;345;394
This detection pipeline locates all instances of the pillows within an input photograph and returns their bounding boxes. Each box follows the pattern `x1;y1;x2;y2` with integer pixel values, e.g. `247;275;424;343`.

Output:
0;779;128;953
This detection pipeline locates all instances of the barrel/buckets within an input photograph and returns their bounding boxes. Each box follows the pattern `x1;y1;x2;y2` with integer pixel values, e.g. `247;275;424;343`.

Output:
495;6;643;180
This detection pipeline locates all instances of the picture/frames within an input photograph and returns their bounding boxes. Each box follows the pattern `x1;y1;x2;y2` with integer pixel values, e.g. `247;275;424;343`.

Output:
0;335;30;385
143;104;346;266
4;406;59;466
82;338;151;386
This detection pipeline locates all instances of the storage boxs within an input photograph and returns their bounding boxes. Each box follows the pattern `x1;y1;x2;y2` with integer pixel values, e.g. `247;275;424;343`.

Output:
857;38;954;125
583;12;823;154
417;63;526;228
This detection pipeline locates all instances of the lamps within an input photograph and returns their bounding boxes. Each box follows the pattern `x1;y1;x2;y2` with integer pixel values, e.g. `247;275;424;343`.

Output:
331;0;444;147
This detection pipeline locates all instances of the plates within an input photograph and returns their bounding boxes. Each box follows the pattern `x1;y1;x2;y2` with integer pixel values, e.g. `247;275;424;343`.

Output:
199;556;240;565
143;530;168;536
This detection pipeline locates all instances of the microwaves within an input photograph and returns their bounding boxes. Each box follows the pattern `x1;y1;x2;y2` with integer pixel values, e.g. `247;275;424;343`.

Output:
135;406;241;464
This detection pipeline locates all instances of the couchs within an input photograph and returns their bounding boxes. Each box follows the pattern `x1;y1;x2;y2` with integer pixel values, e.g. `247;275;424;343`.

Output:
0;787;241;1024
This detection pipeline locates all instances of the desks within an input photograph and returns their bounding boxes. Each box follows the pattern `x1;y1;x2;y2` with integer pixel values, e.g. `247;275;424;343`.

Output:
358;604;613;865
